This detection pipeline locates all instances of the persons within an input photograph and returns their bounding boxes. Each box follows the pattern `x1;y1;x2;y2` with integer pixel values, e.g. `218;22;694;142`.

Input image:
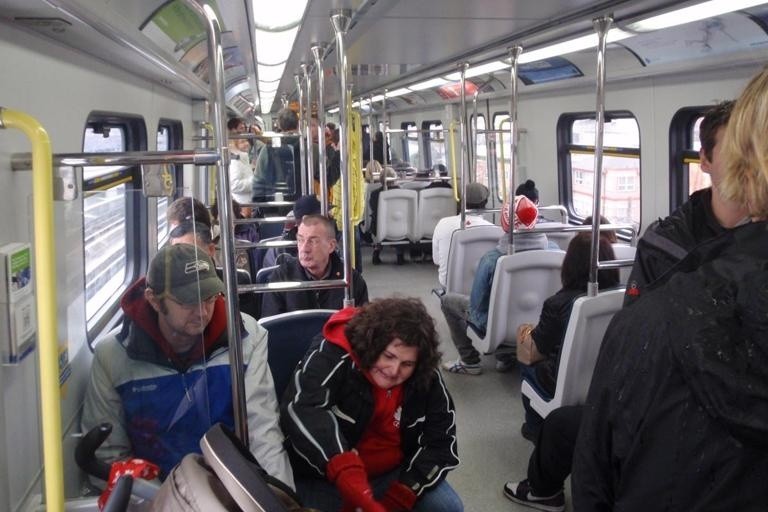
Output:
167;196;453;318
503;404;585;511
515;179;555;223
569;67;767;512
583;214;617;244
279;294;465;511
520;230;620;447
80;242;298;494
431;182;495;292
227;107;397;246
439;196;562;375
427;163;452;190
620;100;750;311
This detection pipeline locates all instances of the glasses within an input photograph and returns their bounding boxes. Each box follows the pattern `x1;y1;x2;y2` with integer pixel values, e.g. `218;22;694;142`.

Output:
164;292;219;310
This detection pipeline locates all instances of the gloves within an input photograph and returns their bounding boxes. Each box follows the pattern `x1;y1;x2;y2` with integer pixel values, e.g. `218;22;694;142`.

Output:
357;479;416;511
326;453;386;511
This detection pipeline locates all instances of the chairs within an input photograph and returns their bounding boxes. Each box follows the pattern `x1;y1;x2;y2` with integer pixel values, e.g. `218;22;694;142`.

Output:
359;160;461;248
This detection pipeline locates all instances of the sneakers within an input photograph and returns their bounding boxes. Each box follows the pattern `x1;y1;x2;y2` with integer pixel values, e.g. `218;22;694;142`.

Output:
373;255;382;265
397;256;408;265
410;256;423;265
503;479;567;512
442;353;483;375
522;422;538;446
494;355;519;373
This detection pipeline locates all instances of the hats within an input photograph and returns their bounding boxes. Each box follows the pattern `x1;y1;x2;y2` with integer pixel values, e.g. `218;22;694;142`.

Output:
146;243;225;306
501;195;539;233
294;195;337;219
466;183;490;203
428;164;448;174
